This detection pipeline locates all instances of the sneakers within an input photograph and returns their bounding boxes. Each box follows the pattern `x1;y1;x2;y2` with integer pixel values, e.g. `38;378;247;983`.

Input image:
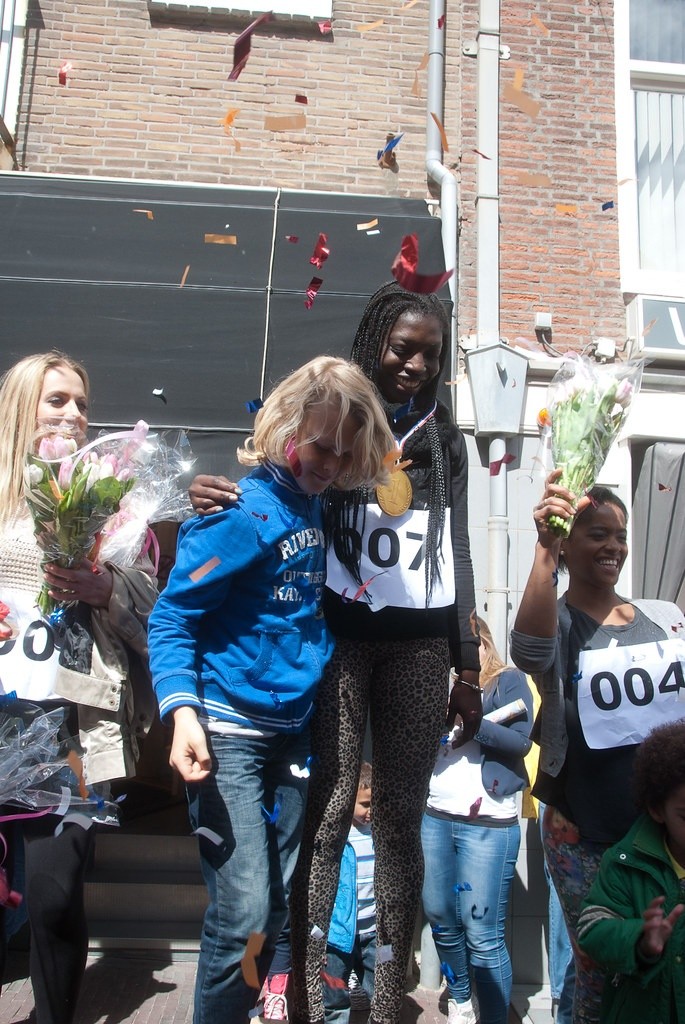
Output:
445;992;481;1024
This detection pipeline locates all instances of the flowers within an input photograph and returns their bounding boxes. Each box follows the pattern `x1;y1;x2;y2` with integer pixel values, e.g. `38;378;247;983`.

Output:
540;354;647;538
23;422;190;627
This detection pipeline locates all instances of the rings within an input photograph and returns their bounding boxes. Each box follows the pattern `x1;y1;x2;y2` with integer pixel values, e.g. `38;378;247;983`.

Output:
470;710;477;714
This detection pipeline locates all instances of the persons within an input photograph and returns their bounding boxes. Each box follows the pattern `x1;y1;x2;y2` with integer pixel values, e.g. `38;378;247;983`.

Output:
538;803;574;1024
319;762;376;1024
576;720;685;1024
262;922;292;1024
0;350;159;1024
150;356;396;1024
507;467;685;1024
188;280;484;1024
422;618;533;1024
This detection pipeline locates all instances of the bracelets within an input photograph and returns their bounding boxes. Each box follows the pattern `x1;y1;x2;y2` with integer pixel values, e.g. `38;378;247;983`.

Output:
455;679;484;693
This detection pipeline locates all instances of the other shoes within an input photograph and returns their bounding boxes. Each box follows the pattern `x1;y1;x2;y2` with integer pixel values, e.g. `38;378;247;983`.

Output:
263;975;290;1024
